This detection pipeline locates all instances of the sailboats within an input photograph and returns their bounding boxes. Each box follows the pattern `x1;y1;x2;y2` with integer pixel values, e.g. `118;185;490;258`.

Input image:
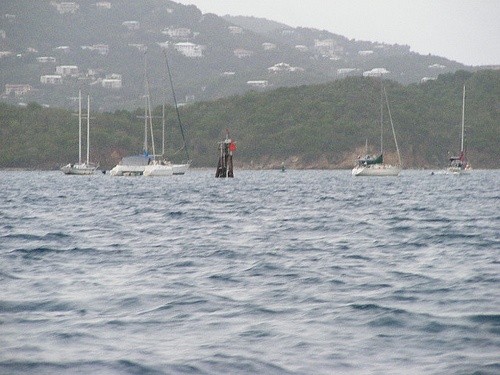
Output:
61;89;99;174
351;79;402;176
432;85;472;174
109;51;191;177
